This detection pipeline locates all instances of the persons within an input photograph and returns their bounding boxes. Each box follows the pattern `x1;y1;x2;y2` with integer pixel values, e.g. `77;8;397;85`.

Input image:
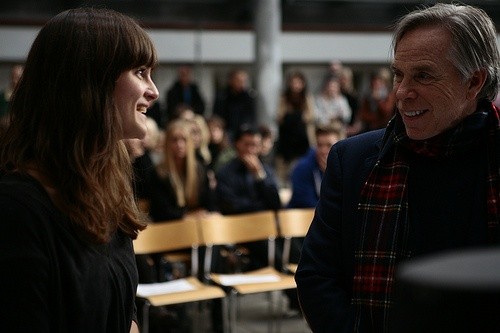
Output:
295;3;500;333
0;8;159;333
5;61;396;285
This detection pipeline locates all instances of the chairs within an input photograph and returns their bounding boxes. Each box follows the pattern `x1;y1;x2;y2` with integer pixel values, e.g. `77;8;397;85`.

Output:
131;217;229;333
200;209;298;333
277;207;316;321
